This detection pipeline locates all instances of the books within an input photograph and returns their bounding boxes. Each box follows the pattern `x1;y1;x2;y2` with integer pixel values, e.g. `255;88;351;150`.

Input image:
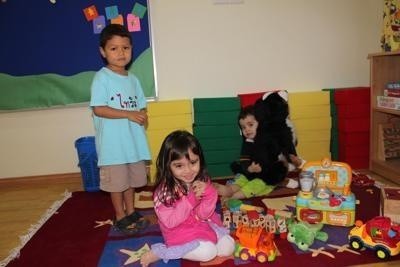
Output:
375;81;400;161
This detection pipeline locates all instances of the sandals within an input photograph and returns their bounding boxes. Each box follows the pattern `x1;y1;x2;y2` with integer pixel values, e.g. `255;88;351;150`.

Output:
127;211;147;228
113;216;138;234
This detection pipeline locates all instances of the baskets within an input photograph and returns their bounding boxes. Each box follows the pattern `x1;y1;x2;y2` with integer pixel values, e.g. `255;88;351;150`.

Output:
74;136;100;192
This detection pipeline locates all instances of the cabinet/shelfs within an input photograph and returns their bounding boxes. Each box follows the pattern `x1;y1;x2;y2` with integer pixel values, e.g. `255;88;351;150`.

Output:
367;51;399;188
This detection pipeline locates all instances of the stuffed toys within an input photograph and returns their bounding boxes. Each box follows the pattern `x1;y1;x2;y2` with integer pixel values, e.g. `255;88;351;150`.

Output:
230;89;303;189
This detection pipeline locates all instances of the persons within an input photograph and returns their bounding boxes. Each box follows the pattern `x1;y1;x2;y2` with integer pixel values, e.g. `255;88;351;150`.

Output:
139;129;235;266
89;23;152;234
211;104;290;202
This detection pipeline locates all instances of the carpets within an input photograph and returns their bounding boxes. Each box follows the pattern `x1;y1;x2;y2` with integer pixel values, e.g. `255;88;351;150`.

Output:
1;170;400;266
